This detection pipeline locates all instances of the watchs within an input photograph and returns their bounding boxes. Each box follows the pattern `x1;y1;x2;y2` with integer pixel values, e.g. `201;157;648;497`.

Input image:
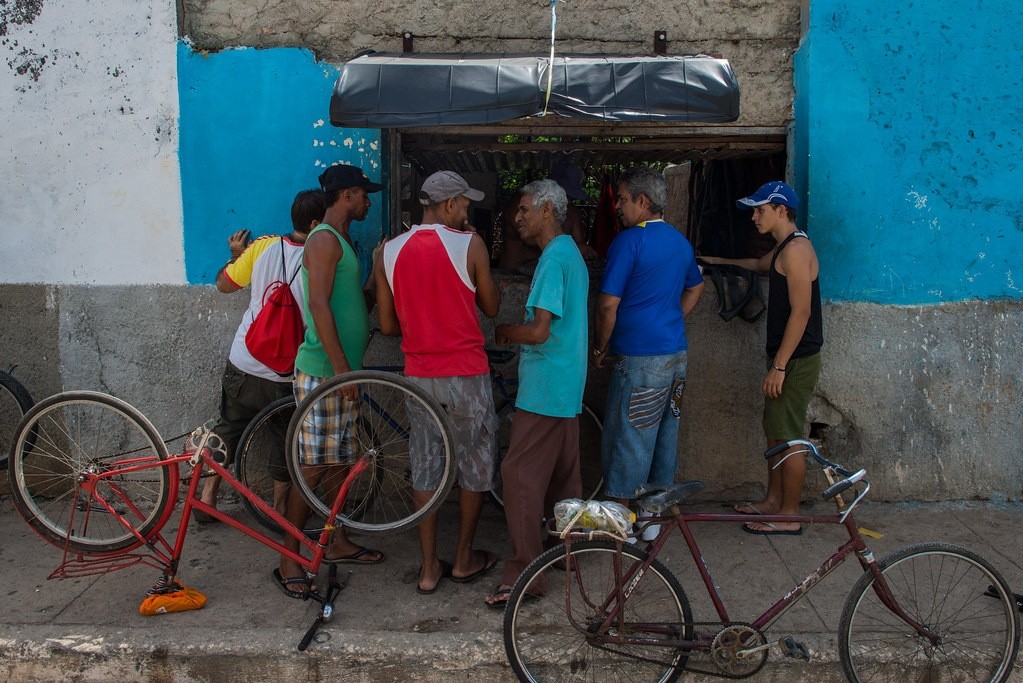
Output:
594;348;606;356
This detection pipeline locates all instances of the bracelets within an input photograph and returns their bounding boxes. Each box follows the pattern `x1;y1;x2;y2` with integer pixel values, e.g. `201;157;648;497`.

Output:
772;364;785;371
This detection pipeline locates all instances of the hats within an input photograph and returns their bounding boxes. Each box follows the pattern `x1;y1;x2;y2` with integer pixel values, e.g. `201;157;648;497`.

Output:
418;171;485;205
319;164;385;194
551;161;585;198
737;181;799;209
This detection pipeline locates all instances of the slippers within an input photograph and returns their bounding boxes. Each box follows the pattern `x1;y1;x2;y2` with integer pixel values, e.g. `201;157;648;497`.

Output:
322;545;385;564
273;567;317;600
742;522;802;535
417;560;449;595
450;548;497;584
485;585;543;607
734;503;774;515
541;539;577;572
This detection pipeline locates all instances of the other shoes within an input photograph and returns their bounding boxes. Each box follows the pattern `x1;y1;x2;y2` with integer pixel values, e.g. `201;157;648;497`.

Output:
192;506;215;523
635;508;660;541
624;530;637;544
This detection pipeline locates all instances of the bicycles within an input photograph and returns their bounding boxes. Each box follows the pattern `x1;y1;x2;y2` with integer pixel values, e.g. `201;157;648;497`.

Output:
8;369;459;653
502;439;1023;683
234;326;605;539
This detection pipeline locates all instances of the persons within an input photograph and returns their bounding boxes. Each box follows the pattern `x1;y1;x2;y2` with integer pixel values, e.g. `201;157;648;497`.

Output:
374;170;502;595
696;181;824;534
193;189;387;522
483;179;589;607
497;163;598;270
588;167;705;544
271;165;387;598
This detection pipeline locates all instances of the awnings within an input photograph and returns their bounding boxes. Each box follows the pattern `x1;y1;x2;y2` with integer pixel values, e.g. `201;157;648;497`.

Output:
328;52;740;129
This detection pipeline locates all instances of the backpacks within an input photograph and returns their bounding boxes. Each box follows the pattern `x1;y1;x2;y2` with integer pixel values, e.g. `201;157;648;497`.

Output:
245;237;303;374
